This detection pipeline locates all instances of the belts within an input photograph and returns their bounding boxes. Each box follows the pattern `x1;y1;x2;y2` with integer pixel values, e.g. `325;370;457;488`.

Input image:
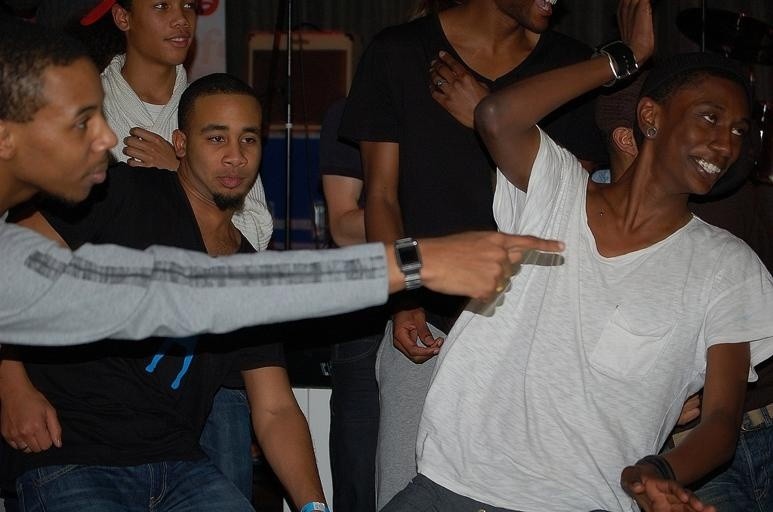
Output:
669;403;773;451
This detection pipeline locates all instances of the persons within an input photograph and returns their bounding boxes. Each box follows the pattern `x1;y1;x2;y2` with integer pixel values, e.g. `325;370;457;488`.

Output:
1;0;772;512
